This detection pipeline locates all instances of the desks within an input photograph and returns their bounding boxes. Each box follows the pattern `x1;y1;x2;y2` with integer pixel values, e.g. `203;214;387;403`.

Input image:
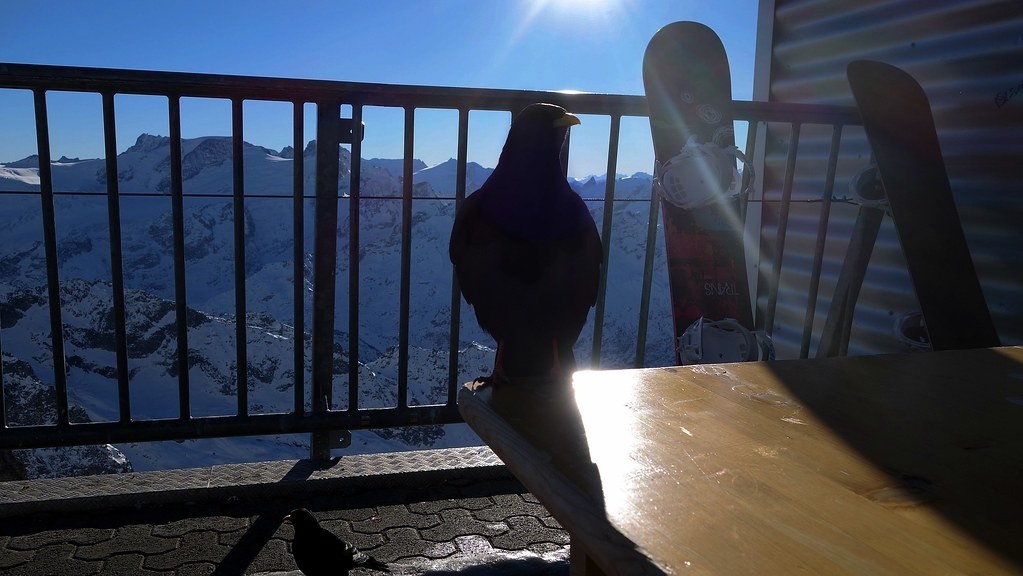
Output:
453;346;1023;576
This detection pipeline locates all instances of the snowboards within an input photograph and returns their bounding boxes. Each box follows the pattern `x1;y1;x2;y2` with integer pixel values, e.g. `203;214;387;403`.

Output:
641;19;777;366
848;59;1005;352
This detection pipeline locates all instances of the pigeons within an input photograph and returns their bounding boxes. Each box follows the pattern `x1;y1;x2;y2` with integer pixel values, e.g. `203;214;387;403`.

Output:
281;507;392;576
